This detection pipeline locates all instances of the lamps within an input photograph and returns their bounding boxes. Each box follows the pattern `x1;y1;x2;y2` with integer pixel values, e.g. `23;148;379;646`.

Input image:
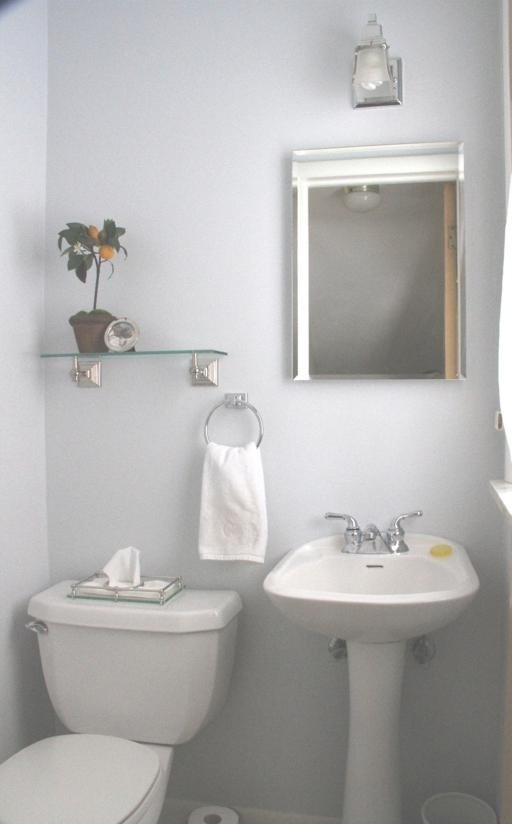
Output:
351;13;402;108
341;185;382;213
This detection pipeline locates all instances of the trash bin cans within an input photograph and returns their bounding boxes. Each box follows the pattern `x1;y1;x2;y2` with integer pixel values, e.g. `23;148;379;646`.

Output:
420;792;497;823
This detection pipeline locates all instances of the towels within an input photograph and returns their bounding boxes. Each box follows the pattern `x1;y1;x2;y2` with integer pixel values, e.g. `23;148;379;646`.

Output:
197;441;269;565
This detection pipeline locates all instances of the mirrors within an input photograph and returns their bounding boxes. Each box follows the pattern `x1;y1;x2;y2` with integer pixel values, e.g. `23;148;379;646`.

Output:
290;141;468;381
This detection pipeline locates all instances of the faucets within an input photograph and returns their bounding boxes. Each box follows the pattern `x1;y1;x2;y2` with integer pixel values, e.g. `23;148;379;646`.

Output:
365;522;401;553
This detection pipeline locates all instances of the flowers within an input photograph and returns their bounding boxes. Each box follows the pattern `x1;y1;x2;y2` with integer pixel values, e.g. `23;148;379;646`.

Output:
57;221;126;317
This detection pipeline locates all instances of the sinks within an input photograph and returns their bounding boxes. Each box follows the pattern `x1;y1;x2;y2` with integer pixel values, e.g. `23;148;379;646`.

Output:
262;534;481;639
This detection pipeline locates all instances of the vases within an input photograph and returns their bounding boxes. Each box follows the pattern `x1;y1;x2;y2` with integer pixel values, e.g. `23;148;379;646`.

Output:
68;317;115;353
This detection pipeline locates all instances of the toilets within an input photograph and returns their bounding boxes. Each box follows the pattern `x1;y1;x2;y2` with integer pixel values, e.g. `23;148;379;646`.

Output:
0;572;243;824
